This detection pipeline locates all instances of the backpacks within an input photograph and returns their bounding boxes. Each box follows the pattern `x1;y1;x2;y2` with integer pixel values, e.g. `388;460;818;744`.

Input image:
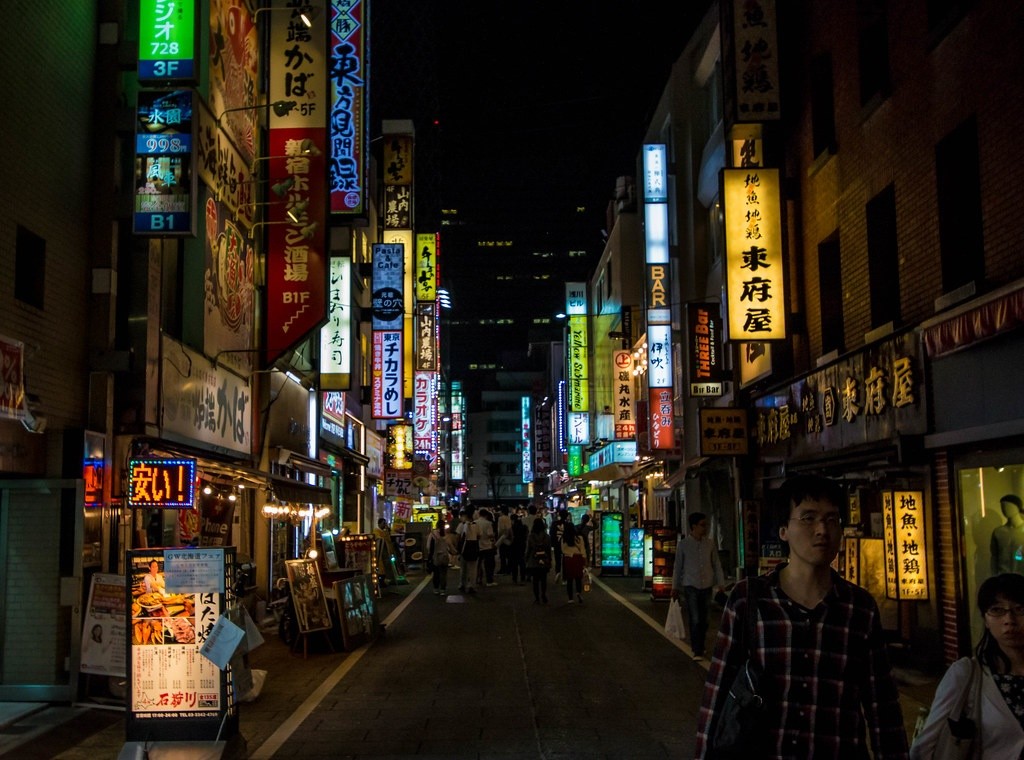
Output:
431;531;449;563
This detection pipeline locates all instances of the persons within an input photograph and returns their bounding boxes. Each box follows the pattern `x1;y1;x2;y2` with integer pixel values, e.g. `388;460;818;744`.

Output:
372;518;394;556
909;572;1024;760
84;625;111;665
670;512;726;661
696;474;910;760
989;492;1024;573
426;504;599;604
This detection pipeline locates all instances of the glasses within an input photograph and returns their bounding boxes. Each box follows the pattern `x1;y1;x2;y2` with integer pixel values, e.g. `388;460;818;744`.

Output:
986;606;1024;617
698;523;710;528
790;515;842;526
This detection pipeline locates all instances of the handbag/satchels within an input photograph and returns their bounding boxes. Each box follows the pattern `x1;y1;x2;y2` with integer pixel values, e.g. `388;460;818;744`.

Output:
664;597;686;640
583;568;591;593
710;576;777;759
913;658;983;760
714;591;728;606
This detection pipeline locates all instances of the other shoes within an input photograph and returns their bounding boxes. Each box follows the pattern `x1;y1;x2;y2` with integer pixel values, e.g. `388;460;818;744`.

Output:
693;647;708;660
560;582;565;586
568;599;574;604
533;599;540;605
526;579;531;583
440;590;445;595
554;573;561;584
468;587;476;597
577;593;584;601
520;578;525;582
542;599;547;605
433;589;439;594
494;571;505;576
486;582;498;586
511;582;517;586
458;585;466;590
